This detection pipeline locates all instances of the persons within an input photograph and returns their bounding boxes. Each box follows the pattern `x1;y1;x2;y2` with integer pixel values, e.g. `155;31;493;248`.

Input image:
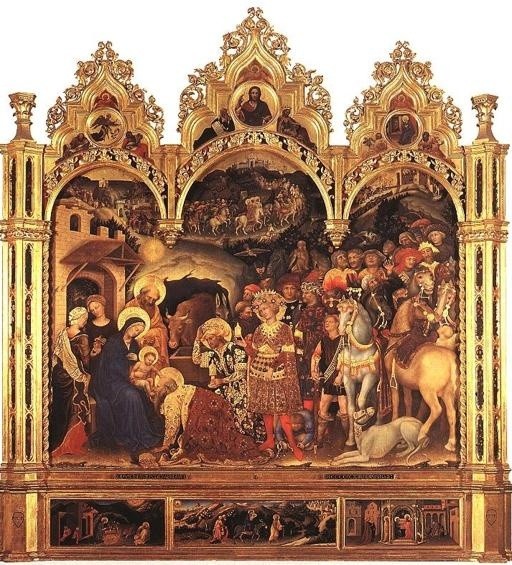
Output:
368;132;388;150
417;131;455;168
403;514;412;539
54;133;90;163
210;514;228;544
94;517;110;545
268;513;283;544
133;521;152;545
243;509;258;530
237;85;271;125
122;130;153;163
277;108;316;151
399;113;413;141
191;108;235;151
91;113;118;140
52;176;461;464
382;516;389;543
